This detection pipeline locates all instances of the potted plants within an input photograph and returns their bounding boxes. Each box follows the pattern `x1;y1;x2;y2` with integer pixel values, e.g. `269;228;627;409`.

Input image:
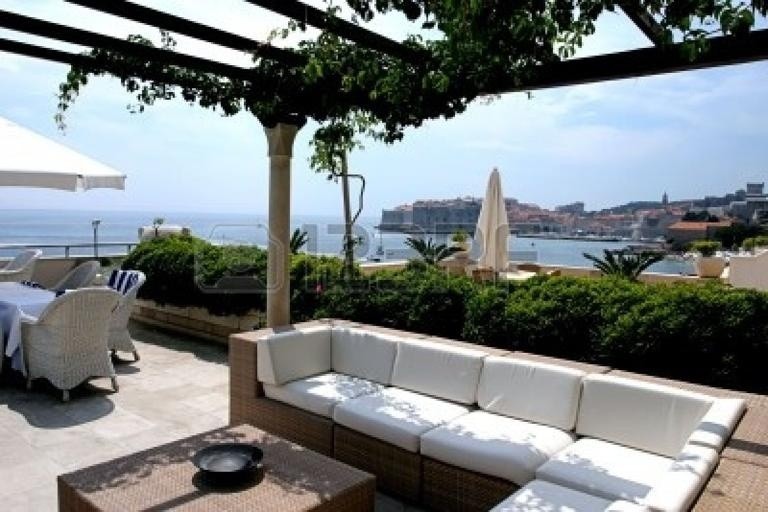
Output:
694;239;725;277
450;229;473;257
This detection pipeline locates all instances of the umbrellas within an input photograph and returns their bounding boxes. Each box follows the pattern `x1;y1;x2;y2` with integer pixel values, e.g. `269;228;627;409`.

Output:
470;166;510;284
0;113;129;195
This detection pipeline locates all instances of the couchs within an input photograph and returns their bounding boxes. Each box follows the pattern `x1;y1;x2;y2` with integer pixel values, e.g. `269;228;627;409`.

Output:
226;317;768;512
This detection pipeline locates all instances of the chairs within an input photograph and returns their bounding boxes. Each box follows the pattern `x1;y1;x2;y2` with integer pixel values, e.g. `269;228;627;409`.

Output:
0;249;146;403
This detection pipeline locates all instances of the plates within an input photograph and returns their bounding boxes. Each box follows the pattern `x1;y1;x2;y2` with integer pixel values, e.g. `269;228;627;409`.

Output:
191;442;263;475
